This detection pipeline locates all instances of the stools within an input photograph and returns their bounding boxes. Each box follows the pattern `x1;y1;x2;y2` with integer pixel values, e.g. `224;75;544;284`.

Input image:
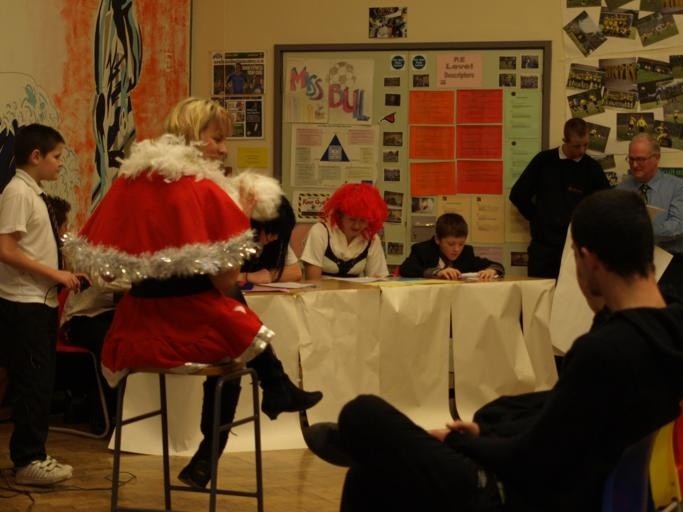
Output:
111;362;264;512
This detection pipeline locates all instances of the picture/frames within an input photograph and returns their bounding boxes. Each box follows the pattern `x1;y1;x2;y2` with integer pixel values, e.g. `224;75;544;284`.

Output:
274;41;551;280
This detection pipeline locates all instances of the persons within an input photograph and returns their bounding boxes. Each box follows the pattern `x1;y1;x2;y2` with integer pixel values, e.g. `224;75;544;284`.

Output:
236;196;303;285
567;63;683;147
46;193;117;435
299;183;390;280
509;118;610;286
0;123;73;486
613;133;682;305
236;101;244;122
400;213;505;282
226;63;249;94
603;14;675;42
305;187;682;511
61;97;322;491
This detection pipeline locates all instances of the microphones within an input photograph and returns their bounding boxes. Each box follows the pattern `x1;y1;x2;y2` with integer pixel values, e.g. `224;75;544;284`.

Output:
56;278;89;289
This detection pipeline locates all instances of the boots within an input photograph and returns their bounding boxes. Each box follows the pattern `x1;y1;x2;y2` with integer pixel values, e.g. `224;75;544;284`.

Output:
176;381;241;491
246;345;323;419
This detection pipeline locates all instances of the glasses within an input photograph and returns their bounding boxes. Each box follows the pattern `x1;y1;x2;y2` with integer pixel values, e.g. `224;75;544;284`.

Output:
625;154;656;164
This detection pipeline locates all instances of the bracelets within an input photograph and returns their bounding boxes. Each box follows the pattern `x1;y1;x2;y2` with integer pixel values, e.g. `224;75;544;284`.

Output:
245;272;248;283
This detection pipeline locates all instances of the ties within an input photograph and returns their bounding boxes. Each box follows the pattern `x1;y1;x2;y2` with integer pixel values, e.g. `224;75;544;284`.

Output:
638;185;650;205
40;191;63;289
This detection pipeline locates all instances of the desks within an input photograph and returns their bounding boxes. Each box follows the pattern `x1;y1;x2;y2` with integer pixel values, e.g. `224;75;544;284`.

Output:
107;274;561;457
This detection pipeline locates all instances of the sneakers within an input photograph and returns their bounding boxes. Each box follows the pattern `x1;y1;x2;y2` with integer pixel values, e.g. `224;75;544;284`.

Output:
15;452;74;487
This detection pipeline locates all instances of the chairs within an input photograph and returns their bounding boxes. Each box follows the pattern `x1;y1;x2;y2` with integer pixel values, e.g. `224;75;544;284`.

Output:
45;287;109;439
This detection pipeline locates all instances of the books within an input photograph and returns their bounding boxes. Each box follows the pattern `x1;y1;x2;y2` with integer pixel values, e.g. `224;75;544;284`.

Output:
646;205;666;223
